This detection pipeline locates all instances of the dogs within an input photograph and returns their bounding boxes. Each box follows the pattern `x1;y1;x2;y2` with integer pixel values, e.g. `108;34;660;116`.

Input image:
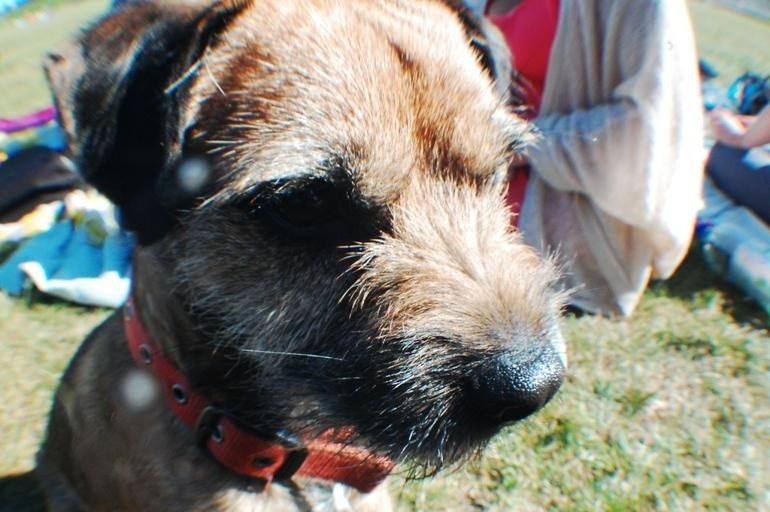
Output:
0;0;590;511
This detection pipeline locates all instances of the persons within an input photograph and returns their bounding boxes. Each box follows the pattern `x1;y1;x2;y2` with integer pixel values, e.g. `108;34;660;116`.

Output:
705;99;770;226
466;0;709;316
